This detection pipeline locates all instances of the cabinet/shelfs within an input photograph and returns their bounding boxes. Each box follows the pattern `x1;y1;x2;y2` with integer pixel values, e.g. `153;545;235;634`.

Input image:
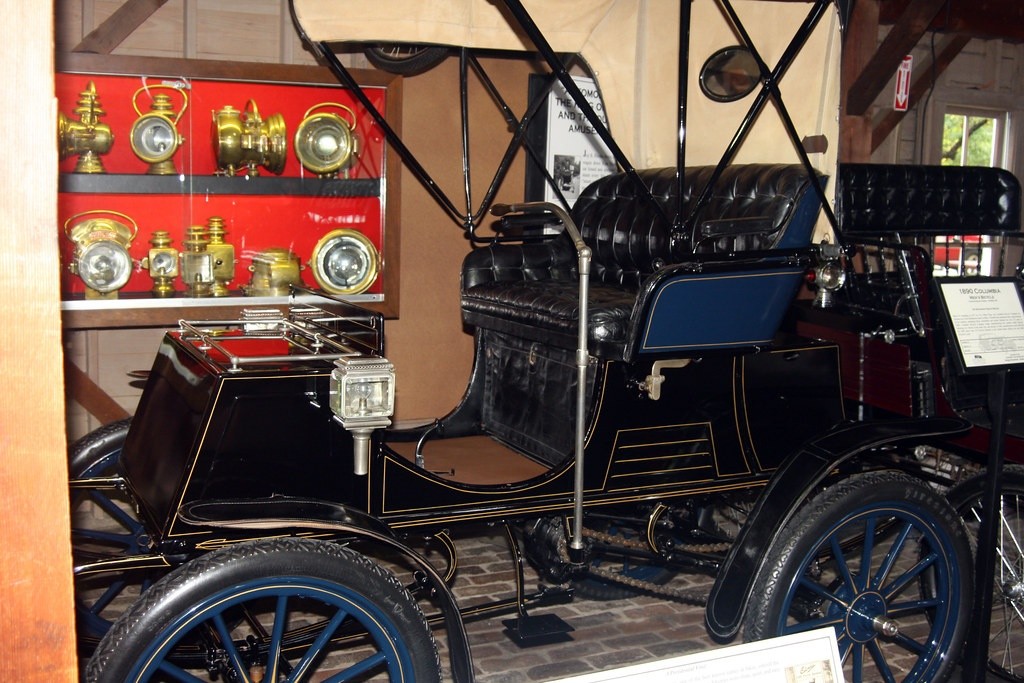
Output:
52;57;400;328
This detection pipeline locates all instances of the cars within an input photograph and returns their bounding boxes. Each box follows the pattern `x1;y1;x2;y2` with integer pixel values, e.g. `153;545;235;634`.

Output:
933;233;983;277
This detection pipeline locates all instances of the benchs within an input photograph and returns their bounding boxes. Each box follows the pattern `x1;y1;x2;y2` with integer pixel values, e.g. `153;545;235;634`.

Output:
460;165;830;363
832;160;1022;326
